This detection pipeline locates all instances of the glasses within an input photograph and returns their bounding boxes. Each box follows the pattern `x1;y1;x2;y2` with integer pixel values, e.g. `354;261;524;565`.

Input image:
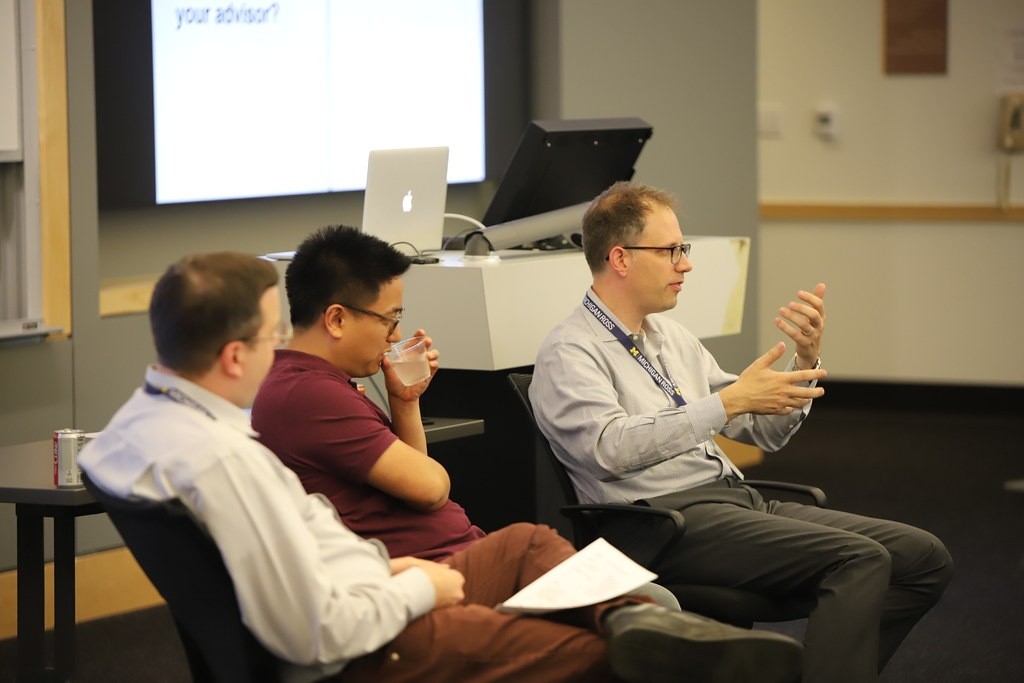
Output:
323;302;406;337
605;244;691;265
216;320;289;360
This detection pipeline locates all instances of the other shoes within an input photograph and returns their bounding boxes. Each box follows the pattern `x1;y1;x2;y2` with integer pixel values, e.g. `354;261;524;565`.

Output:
604;603;806;683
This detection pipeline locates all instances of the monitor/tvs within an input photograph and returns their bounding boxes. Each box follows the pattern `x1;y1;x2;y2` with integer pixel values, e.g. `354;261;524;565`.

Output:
483;117;654;229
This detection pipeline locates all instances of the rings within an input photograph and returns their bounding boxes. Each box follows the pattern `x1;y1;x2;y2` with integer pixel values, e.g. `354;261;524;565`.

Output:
801;326;814;336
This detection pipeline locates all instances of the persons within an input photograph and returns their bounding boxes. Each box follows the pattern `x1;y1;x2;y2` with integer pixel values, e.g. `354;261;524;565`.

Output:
248;225;488;564
79;251;806;682
527;180;950;683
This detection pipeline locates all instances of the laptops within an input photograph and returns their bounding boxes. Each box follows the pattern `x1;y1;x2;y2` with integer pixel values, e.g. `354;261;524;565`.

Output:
267;146;451;261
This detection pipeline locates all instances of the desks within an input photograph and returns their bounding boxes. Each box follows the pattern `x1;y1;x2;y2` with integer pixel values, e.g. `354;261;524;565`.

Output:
0;411;484;683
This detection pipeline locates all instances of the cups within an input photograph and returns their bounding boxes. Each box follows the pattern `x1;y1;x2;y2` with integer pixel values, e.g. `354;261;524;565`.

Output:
383;336;432;386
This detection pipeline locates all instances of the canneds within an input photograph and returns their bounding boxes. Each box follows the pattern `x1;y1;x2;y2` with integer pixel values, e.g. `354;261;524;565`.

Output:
53;428;85;488
357;384;365;394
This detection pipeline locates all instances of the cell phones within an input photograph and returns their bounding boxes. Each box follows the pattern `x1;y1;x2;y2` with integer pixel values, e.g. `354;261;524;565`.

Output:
409;257;439;266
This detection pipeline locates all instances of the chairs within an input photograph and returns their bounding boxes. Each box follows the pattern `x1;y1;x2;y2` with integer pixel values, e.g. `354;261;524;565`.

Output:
507;371;827;632
78;471;366;683
